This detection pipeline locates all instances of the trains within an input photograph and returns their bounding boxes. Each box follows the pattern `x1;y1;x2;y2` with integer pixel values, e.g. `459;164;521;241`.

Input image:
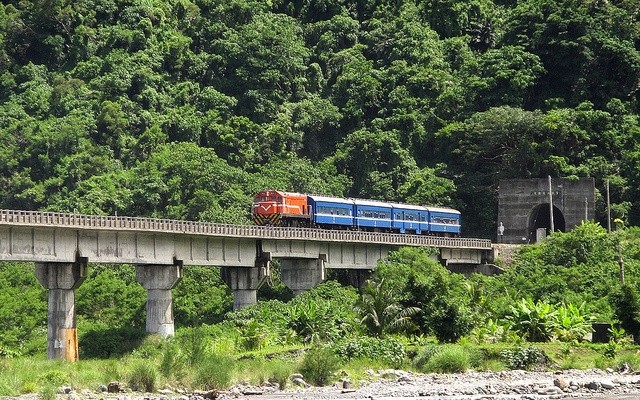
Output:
251;190;461;238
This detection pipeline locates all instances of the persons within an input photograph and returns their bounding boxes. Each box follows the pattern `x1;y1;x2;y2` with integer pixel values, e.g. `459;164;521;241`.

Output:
496;220;505;242
